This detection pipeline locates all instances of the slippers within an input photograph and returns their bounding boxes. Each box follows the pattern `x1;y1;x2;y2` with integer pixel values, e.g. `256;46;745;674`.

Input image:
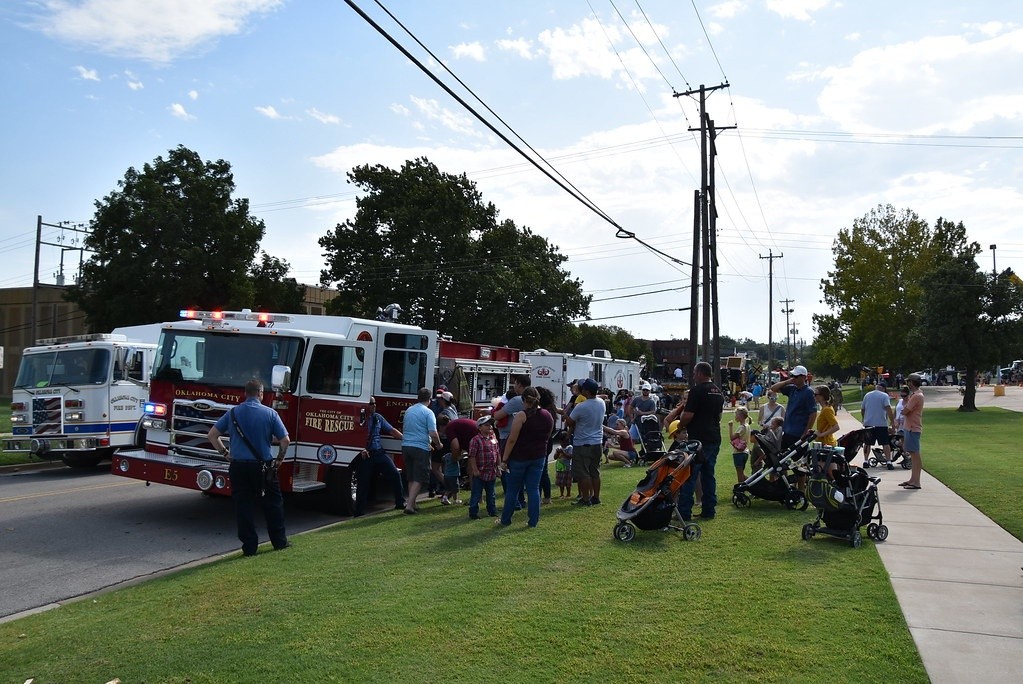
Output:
898;482;922;489
394;503;420;514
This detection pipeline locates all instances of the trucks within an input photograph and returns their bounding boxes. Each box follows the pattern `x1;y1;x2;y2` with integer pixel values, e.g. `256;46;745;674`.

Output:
1000;360;1023;384
908;368;954;386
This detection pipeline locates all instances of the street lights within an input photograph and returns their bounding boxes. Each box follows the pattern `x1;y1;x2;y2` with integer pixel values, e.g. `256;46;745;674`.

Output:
989;243;997;281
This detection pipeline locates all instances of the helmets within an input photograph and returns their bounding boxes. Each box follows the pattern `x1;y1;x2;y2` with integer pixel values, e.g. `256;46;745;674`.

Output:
668;420;681;440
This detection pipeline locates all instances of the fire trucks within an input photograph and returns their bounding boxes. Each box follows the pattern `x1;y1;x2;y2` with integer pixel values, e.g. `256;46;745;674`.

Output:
111;309;533;517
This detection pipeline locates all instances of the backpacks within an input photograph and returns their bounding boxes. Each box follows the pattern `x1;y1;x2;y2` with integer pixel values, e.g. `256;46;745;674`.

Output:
804;448;846;514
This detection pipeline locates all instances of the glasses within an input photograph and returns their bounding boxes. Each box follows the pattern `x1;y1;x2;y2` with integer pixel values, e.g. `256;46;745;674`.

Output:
901;393;909;397
370;401;376;405
789;374;803;378
769;397;778;401
814;393;821;397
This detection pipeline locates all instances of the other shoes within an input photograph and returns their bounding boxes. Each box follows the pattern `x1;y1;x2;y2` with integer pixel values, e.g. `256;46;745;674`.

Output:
469;512;503;525
672;509;715;522
571;494;600;507
441;496;462;505
284;541;292;548
863;459;870;468
541;498;552;505
886;460;894;470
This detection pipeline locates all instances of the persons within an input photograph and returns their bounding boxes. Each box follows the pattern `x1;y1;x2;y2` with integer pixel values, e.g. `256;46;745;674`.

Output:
352;395;406;517
861;370;923;489
208;379;294;557
428;355;845;527
401;388;443;515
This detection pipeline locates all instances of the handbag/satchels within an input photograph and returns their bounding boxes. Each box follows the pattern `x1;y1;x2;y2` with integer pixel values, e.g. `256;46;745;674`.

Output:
730;424;747;451
258;466;281;514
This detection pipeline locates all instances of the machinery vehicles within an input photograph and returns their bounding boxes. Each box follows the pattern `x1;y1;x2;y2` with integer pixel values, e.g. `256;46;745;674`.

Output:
643;339;766;410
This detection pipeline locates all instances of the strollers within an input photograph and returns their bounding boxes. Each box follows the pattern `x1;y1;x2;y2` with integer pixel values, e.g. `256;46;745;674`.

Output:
612;439;702;543
868;430;912;470
634;413;668;467
732;429;816;511
802;448;890;549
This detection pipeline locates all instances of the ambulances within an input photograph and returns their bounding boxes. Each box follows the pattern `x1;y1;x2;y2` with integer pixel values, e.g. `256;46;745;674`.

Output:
519;349;662;431
9;320;206;471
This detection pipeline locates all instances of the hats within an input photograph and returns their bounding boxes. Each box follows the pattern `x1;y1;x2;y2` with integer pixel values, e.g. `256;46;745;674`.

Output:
566;378;598;392
642;383;652;391
904;373;921;381
435;384;453;402
877;382;886;392
476;417;495;429
790;365;808;377
